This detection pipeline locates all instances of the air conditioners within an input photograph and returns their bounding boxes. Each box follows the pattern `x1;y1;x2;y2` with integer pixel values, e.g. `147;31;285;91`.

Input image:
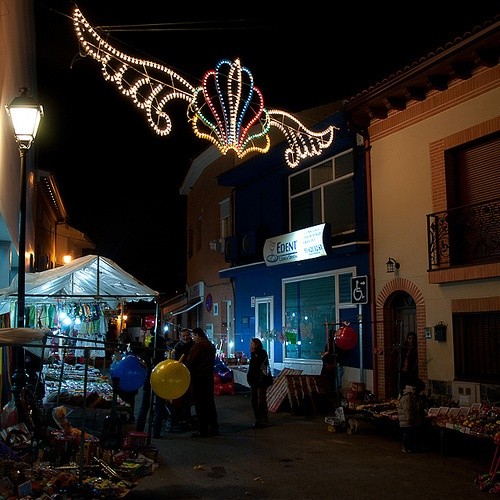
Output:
38;254;50;271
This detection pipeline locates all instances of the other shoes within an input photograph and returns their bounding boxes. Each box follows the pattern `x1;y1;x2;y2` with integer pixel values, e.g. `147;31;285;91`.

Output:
401;449;408;453
253;423;267;429
191;432;201;438
154;433;166;439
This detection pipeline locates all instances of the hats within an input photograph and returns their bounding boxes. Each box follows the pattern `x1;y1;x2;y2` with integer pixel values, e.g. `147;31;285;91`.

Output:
190;328;203;334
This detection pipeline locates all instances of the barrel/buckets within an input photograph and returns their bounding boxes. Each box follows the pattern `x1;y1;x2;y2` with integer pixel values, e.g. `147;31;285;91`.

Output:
352;383;365;391
349;402;364;409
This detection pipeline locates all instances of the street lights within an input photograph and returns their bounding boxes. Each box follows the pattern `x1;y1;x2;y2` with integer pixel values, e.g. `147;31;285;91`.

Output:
4;85;45;430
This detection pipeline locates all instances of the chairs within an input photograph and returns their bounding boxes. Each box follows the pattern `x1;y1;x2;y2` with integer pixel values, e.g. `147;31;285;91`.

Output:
17;388;54;461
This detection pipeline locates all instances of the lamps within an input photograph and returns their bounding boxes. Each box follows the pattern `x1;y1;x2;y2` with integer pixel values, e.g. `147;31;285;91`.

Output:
386;257;400;273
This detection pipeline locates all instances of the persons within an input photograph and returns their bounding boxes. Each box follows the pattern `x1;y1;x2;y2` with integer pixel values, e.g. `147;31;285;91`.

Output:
401;332;418;390
126;328;220;439
247;337;273;431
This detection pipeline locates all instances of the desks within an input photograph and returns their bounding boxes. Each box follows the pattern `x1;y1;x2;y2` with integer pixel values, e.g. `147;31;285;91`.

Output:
227;364;251;397
434;420;492;456
42;397;132;429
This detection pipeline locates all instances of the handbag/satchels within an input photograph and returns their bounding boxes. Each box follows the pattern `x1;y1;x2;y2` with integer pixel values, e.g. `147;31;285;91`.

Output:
267;370;274;388
401;346;410;372
